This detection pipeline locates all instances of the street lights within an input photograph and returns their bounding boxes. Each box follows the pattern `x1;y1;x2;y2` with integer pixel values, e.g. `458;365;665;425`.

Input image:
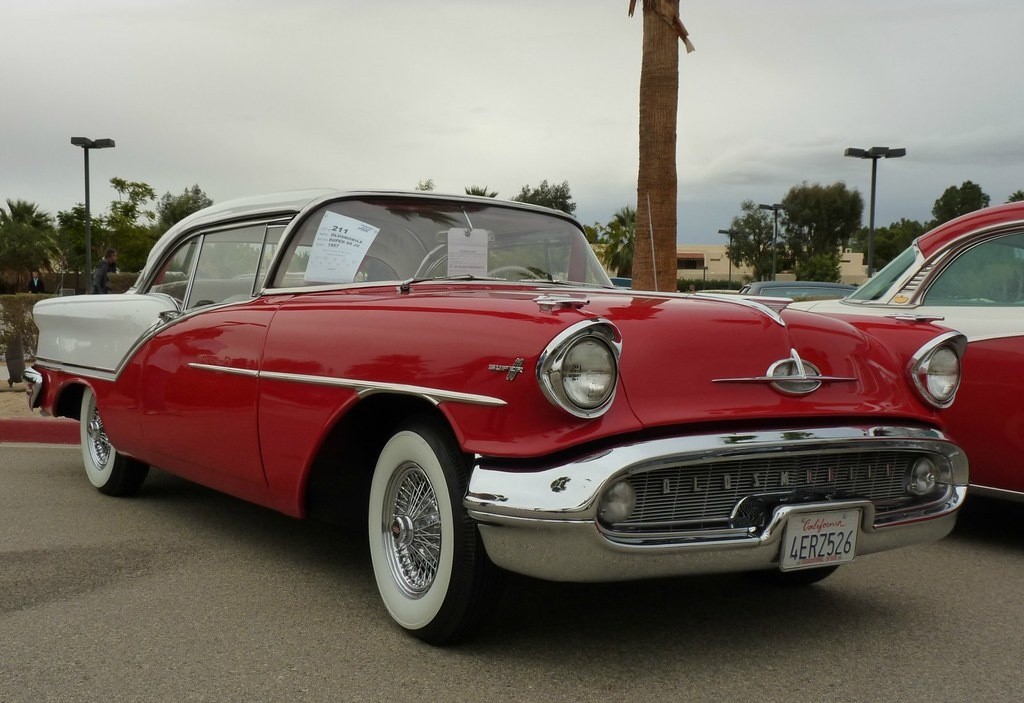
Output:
718;228;739;289
758;203;785;280
843;145;906;278
69;135;118;296
700;266;708;290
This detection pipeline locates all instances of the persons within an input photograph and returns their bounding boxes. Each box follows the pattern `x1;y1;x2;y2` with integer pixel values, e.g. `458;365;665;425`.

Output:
92;249;117;294
29;271;45;293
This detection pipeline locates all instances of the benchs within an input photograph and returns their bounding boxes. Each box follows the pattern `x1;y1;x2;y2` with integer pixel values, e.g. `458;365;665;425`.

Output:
151;271;305;311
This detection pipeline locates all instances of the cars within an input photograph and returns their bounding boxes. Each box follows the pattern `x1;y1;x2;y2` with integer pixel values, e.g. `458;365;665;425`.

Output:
18;187;971;649
782;198;1024;507
740;282;858;302
